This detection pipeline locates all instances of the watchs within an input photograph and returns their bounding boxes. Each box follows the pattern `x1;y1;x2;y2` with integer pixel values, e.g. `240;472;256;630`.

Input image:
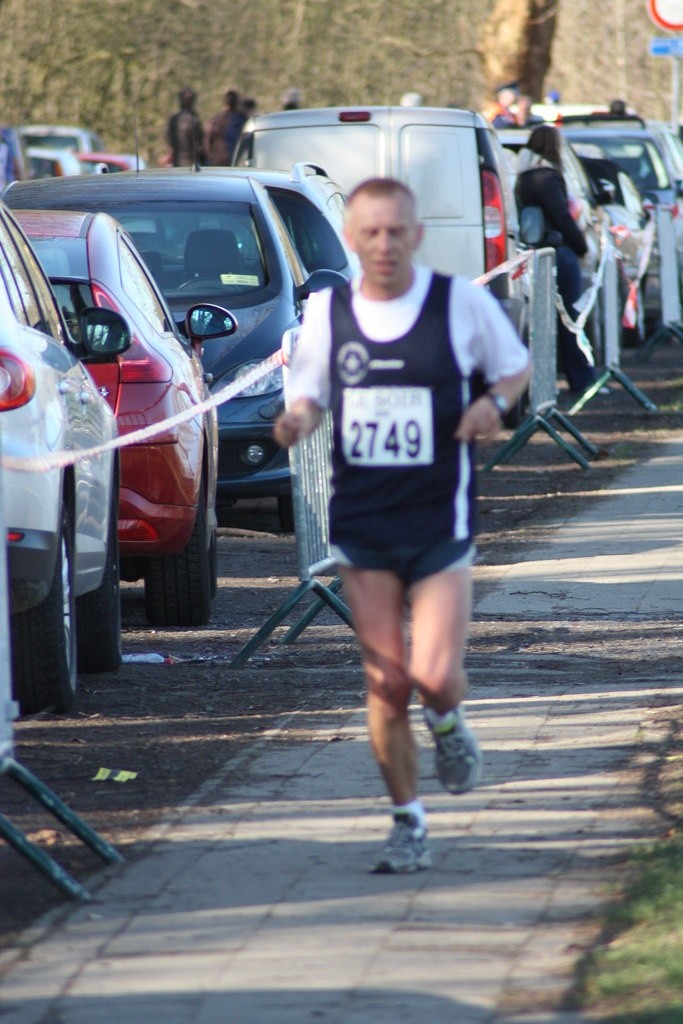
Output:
486;392;508;415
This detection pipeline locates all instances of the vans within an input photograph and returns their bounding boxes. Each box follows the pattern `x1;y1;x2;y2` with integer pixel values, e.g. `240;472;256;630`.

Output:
231;107;521;324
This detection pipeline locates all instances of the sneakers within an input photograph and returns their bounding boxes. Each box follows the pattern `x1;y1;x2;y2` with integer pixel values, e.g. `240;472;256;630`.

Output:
424;706;479;796
367;812;430;873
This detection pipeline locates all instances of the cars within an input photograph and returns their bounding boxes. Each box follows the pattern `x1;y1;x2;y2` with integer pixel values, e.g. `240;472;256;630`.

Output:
0;125;363;713
499;102;682;343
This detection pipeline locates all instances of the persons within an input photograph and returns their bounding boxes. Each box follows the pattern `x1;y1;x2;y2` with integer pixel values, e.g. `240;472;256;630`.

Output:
165;88;206;167
273;178;535;872
610;100;625;116
280;87;300;109
513;125;612;396
485;83;544;128
208;90;257;166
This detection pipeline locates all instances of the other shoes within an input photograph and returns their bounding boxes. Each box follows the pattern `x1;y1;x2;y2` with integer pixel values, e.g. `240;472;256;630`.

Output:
584;384;610;394
